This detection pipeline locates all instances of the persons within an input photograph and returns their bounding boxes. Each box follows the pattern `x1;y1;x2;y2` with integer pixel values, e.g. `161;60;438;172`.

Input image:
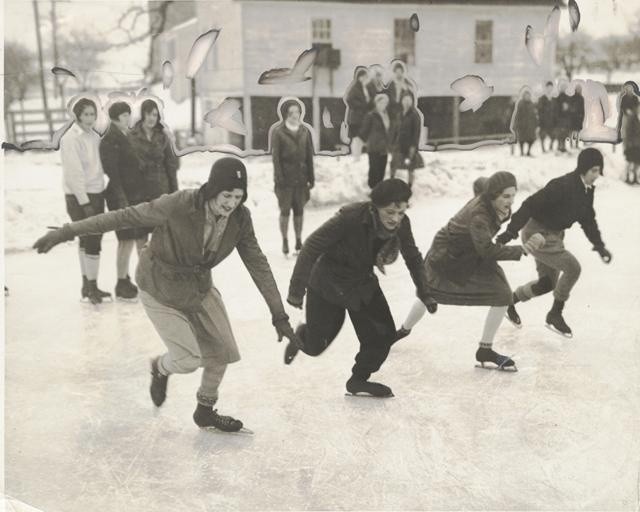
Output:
270;100;314;254
512;91;537;156
348;70;372;161
555;86;572;151
619;85;640;141
366;71;388;108
571;87;584;148
537;81;558;151
620;99;640;184
388;90;424;188
361;94;392;187
393;172;545;367
388;63;416;153
60;99;111;302
33;157;300;430
285;179;437;397
100;101;138;298
127;99;178;257
498;148;611;332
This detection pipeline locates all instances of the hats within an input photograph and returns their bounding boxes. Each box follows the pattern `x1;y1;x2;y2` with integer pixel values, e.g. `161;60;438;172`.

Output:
578;148;605;177
487;171;517;192
211;157;250;188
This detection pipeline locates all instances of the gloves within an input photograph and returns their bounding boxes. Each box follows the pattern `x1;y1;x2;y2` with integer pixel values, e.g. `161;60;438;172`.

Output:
523;233;546;256
31;222;76;254
592;242;611;263
287;296;304;309
421;296;438;313
496;227;518;244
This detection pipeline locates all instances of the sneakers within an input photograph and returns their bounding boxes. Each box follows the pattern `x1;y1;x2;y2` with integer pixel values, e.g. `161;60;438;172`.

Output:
546;312;572;333
81;284;111;297
116;280;136;299
295;241;303;250
283;328;304;364
150;357;169;408
345;377;393;398
192;406;243;432
86;285;102;302
282;237;289;254
507;304;522;324
274;319;305;350
394;328;409;342
476;347;514;366
